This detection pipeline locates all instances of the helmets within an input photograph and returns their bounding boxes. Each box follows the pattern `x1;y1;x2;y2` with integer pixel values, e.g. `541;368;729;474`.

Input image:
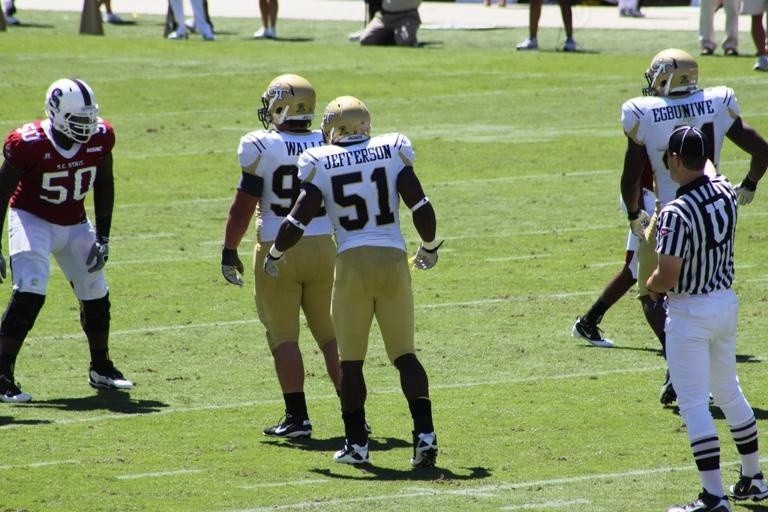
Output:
45;78;98;143
321;96;371;146
259;74;316;129
643;51;698;96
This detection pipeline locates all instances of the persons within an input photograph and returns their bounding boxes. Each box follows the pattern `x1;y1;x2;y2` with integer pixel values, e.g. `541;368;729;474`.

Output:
571;70;657;348
97;0;123;24
646;126;768;512
255;0;279;39
168;1;213;40
360;0;421;47
516;1;576;51
349;0;384;41
741;2;767;71
1;77;135;402
164;1;212;38
0;0;20;31
620;48;768;404
262;96;444;467
222;74;369;439
699;1;739;55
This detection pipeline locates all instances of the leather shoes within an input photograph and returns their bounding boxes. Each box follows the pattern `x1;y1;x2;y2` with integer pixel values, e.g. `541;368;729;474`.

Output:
701;48;714;55
725;48;737;56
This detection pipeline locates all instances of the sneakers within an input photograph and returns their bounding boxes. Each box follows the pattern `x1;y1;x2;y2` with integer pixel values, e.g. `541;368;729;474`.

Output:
107;14;120;22
334;440;369;464
0;376;32;402
265;28;277;37
411;432;438;467
561;40;574;51
731;472;768;501
263;416;310;439
3;16;19;24
571;318;613;347
255;28;266;38
660;370;677;406
516;39;537;49
203;34;214;40
364;422;371;432
669;493;730;511
89;366;133;390
168;30;189;40
754;57;768;70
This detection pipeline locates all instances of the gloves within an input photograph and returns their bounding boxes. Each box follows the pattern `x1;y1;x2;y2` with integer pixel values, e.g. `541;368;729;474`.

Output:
221;247;245;285
263;244;283;278
628;209;651;237
414;243;439;270
0;250;6;284
733;176;757;205
86;239;109;273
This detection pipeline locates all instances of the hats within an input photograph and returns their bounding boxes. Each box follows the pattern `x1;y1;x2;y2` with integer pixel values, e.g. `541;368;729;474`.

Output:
657;127;709;158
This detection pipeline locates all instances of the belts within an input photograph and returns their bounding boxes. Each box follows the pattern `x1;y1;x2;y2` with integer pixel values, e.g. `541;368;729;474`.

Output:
381;8;417;15
